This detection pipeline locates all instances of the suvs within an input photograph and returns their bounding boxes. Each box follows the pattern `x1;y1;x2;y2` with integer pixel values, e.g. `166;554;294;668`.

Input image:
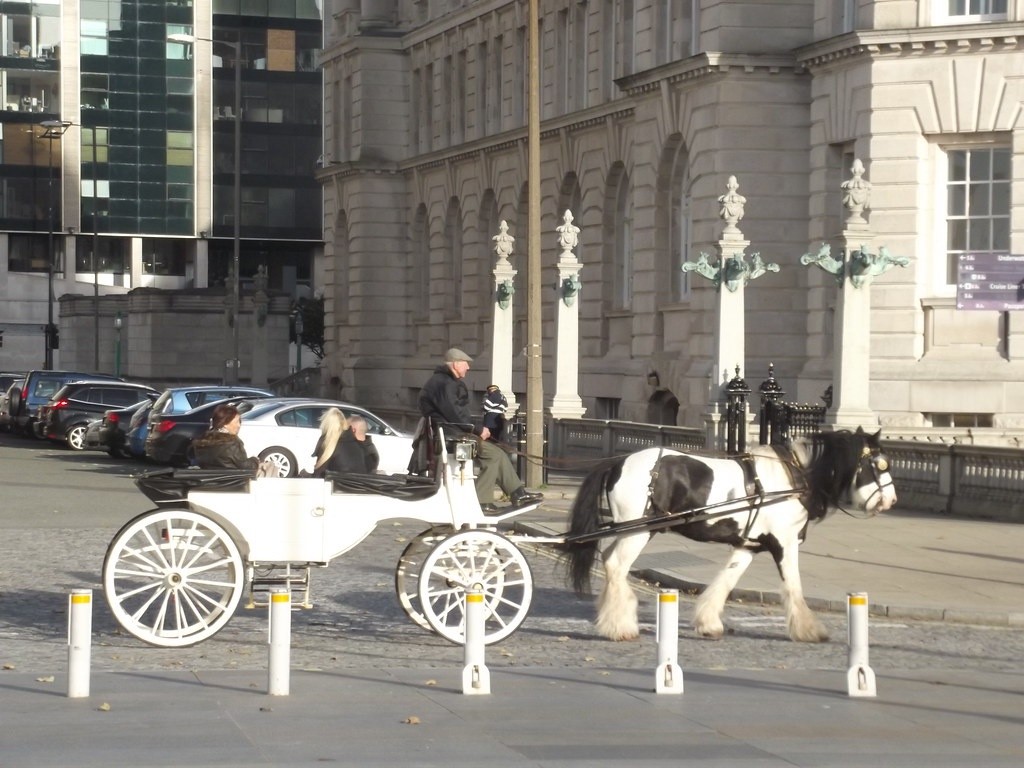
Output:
143;387;309;466
8;370;129;441
43;382;164;451
4;380;42;427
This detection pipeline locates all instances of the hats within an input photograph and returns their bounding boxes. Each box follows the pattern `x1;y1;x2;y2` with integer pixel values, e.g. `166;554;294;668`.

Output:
445;348;474;363
486;385;500;390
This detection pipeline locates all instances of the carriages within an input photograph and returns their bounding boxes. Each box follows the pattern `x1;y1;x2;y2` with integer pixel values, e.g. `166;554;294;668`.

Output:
102;391;897;650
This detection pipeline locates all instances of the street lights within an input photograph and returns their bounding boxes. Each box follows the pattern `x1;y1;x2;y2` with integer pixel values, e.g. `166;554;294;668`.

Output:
27;110;76;374
166;30;245;379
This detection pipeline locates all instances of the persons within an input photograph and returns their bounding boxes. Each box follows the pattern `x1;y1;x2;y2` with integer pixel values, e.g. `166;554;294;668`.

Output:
312;408;368;477
417;347;543;517
190;408;278;479
297;415;380;479
480;384;508;445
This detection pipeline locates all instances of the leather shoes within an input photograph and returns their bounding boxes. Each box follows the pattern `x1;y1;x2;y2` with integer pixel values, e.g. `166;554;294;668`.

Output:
512;488;543;507
480;504;505;515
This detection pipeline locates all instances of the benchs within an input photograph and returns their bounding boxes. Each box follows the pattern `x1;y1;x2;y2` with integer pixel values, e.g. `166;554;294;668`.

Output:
424;416;479;475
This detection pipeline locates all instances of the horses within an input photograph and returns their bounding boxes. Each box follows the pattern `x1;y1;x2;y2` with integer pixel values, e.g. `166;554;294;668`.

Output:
554;426;897;642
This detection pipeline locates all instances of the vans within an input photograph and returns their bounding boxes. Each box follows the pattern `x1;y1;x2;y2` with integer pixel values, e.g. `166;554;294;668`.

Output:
0;372;29;430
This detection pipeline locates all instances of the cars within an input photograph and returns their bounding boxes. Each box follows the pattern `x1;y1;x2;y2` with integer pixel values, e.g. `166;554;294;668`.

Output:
125;393;161;459
216;399;423;479
97;396;151;462
